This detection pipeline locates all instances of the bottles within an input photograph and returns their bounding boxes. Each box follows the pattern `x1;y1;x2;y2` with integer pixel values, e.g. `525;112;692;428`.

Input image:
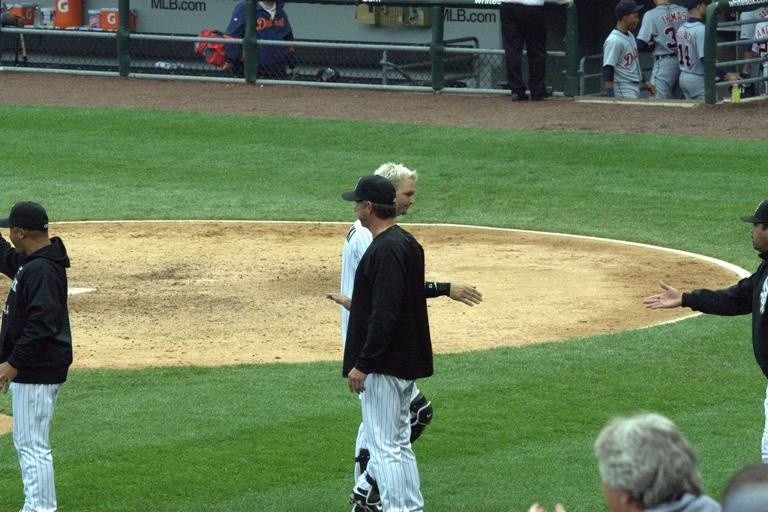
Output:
731;84;741;101
33;7;40;28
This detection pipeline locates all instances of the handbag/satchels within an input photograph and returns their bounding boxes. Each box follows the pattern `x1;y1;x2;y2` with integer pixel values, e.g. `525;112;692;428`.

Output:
196;29;244;67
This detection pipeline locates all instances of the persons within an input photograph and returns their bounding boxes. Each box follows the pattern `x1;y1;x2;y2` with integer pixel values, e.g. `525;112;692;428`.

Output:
601;1;768;102
325;177;435;511
499;0;554;102
642;202;766;467
221;0;301;81
340;162;482;512
0;202;74;512
527;413;768;511
0;4;26;28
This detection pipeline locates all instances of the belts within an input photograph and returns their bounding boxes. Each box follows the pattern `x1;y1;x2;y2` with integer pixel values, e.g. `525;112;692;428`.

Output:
655;54;678;59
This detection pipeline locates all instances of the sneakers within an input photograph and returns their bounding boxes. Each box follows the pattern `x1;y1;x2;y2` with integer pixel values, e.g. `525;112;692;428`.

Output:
350;485;383;511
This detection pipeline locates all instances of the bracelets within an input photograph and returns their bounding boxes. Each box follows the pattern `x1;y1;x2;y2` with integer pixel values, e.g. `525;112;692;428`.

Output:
425;280;450;299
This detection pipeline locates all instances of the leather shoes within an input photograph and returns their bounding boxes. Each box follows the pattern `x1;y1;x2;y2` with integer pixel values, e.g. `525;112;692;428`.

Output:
512;94;529;101
531;86;553;100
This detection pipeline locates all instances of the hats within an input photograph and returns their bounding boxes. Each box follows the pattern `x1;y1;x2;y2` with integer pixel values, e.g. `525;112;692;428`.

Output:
1;202;48;230
341;175;396;204
740;200;768;222
615;1;644;15
